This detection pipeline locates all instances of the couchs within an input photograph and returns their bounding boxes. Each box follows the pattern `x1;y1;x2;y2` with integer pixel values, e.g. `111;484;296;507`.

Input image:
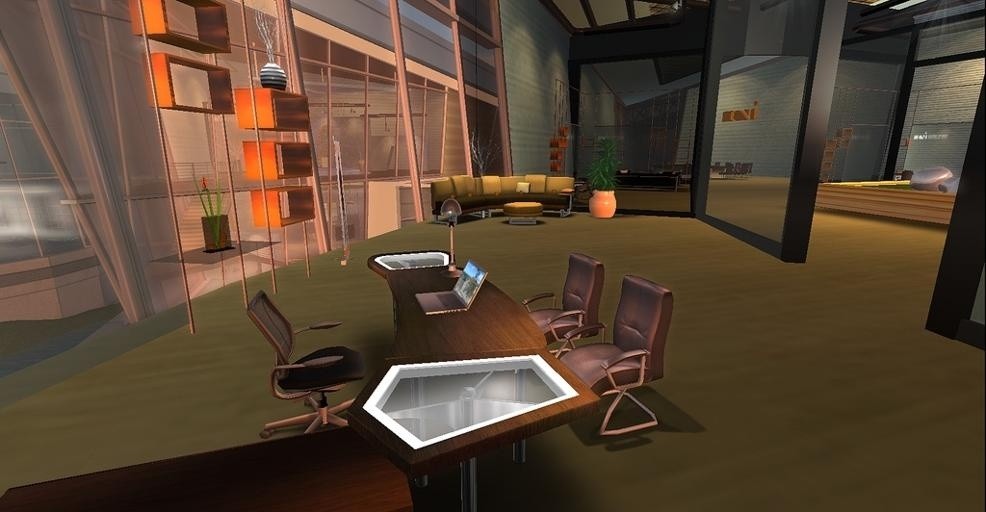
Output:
431;174;575;218
614;168;681;193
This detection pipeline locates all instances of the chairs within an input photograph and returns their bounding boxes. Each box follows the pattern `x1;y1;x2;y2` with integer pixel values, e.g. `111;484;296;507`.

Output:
247;288;366;439
564;274;673;441
711;159;753;177
520;251;604;355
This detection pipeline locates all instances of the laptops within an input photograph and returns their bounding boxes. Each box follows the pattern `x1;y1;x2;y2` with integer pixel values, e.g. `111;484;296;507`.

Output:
415;258;488;315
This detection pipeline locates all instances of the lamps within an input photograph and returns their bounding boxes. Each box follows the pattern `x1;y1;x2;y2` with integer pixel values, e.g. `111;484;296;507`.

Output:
438;198;463;280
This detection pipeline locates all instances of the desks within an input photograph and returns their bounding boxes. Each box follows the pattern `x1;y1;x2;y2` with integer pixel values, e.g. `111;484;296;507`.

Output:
367;248;455;279
502;202;542;225
348;348;601;511
388;263;547;360
1;426;413;512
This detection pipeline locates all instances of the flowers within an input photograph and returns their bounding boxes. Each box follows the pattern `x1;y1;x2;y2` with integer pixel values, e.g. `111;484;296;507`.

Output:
188;167;231;245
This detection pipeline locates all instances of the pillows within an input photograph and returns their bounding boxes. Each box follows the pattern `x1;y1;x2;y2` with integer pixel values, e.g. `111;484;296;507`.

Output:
516;182;530;192
451;175;474;197
525;175;547;193
433;178;453;200
561;188;574;193
481;176;501;194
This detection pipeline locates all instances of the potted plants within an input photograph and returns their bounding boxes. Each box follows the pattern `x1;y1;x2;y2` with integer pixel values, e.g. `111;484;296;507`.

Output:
585;135;621;218
254;8;290;91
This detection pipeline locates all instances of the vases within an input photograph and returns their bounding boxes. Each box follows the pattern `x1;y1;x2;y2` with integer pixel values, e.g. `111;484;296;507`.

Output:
200;215;233;251
